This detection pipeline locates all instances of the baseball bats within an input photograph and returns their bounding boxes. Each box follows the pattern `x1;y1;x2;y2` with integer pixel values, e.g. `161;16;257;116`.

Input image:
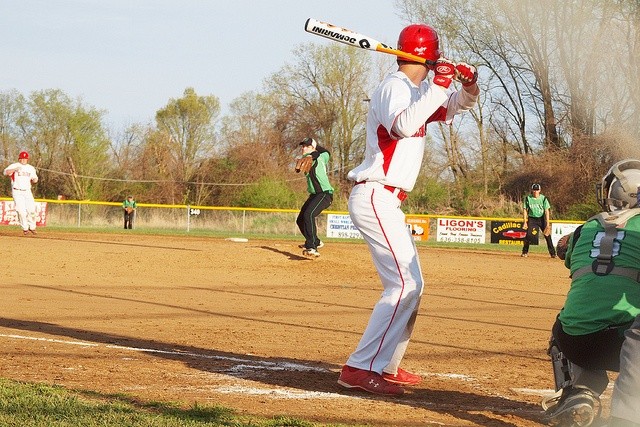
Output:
306;20;434;66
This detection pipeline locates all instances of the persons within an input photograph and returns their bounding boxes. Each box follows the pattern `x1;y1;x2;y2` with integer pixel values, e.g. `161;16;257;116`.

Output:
122;196;137;229
337;24;480;398
538;158;640;427
296;138;334;258
521;184;556;258
3;151;38;235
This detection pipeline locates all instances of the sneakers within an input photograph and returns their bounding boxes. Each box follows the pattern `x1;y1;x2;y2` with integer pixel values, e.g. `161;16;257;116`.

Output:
551;254;558;258
380;366;425;386
537;387;603;426
337;363;404;396
299;239;324;250
30;229;38;234
303;247;320;257
521;252;529;257
23;229;29;234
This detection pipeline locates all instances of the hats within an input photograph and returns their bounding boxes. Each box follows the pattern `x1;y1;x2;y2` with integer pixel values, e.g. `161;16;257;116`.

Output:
531;183;541;190
299;137;317;147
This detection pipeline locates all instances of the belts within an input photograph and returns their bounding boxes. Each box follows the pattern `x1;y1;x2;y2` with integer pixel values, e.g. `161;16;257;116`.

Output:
354;180;407;202
14;187;27;191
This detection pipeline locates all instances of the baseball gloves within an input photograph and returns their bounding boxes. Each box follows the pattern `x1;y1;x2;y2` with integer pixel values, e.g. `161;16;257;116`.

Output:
295;158;312;172
126;206;135;214
556;234;571;259
544;228;552;237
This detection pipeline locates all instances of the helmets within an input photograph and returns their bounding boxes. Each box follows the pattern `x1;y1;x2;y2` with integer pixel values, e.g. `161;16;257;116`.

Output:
19;151;29;159
596;158;640;213
396;24;441;70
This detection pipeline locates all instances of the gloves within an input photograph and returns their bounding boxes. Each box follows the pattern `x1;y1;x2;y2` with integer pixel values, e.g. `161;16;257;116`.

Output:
451;61;479;89
433;50;457;88
